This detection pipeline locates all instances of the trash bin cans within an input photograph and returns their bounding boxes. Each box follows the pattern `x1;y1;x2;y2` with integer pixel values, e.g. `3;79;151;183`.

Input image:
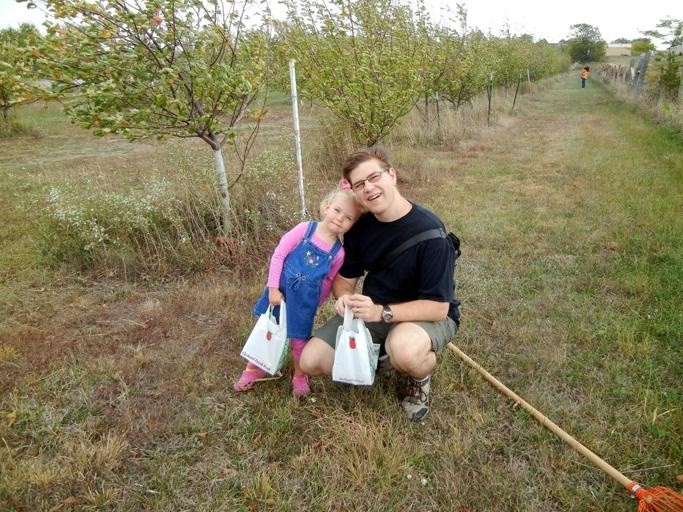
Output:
380;303;394;325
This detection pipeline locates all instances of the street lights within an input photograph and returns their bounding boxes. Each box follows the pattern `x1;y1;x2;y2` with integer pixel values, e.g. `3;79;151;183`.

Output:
241;299;289;376
331;303;380;386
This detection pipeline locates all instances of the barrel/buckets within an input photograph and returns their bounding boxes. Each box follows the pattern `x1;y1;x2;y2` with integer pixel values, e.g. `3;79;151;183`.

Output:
233;369;267;392
291;375;311;396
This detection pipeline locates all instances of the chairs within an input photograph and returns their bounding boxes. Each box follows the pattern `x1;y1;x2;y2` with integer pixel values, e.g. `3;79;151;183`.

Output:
377;354;395;381
401;376;430;421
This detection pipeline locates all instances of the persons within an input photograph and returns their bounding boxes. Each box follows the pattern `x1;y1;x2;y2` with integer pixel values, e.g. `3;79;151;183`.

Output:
298;147;464;426
232;174;370;401
580;66;591;89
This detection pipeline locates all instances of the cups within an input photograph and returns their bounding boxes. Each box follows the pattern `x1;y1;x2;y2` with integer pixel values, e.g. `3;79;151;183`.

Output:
350;169;388;190
